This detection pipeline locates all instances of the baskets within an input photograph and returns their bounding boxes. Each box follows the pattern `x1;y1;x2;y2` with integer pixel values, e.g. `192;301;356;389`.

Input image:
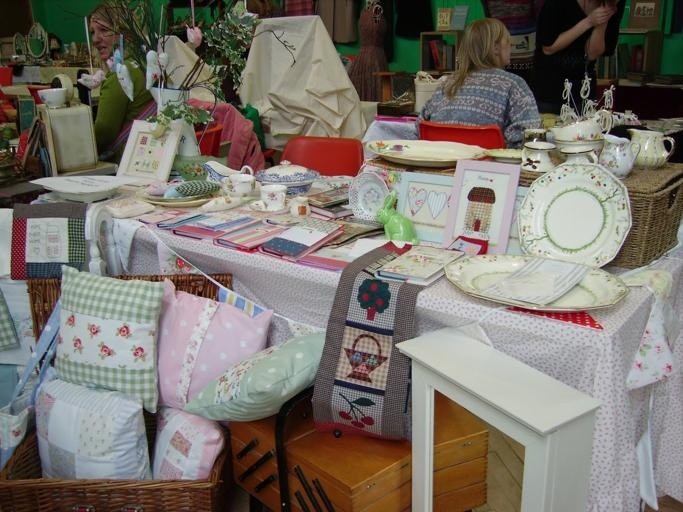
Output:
488;135;681;271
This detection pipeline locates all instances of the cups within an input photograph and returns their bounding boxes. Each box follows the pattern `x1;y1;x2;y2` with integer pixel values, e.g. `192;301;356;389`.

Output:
200;157;318;220
484;116;676;180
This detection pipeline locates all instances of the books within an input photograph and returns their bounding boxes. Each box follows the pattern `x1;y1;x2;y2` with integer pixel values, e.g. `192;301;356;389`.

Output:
427;37;455;69
596;0;683;84
438;4;470;32
140;188;463;285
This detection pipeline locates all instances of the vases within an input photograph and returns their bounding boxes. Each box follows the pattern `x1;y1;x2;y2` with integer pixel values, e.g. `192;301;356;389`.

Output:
150;86;199;157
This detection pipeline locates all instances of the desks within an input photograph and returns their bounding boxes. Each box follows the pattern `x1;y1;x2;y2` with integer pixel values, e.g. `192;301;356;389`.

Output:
9;65;100;86
362;115;416;158
33;194;683;512
0;160;119;209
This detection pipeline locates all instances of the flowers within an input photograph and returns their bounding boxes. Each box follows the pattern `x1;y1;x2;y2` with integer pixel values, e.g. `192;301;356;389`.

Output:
66;0;296;144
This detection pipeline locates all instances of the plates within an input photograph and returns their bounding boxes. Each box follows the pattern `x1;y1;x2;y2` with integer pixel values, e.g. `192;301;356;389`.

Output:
135;187;214;207
519;162;633;270
42;183;122;203
443;251;631;311
367;139;486;169
349;173;392;222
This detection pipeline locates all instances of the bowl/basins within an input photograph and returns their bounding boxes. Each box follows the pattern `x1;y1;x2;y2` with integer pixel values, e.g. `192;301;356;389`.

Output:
36;87;69;109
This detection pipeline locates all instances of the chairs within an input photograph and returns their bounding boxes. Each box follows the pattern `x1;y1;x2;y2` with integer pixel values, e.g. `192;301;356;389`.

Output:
420;122;504;149
196;124;222;156
282;135;362;176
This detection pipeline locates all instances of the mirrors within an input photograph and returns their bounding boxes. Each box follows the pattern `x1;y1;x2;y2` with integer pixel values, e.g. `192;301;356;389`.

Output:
411;357;550;512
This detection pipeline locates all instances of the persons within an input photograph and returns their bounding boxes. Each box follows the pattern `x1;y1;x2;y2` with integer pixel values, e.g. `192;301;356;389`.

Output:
415;17;541;150
89;3;159;154
532;1;626;116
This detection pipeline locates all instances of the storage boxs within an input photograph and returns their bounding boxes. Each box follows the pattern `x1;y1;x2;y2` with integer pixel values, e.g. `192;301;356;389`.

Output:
229;388;412;512
433;389;487;512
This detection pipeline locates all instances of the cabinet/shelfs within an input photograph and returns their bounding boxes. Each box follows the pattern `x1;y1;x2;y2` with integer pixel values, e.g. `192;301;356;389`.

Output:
372;29;683;120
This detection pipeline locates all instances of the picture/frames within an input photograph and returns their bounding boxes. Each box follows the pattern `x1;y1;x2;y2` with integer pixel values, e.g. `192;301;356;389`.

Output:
445;160;519;255
397;172;453;243
